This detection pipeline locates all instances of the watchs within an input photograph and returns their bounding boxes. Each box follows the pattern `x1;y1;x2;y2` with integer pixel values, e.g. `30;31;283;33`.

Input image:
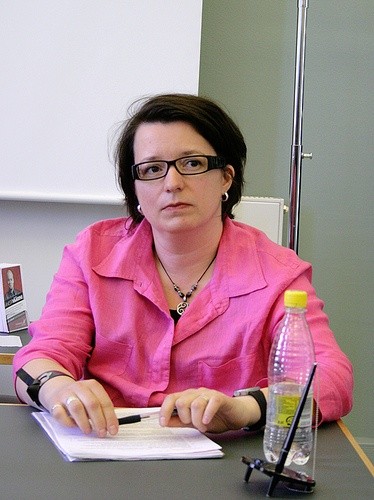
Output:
232;386;267;429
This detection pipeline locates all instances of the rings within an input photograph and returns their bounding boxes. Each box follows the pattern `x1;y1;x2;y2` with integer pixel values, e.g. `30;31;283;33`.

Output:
199;394;210;402
50;404;62;414
65;396;80;407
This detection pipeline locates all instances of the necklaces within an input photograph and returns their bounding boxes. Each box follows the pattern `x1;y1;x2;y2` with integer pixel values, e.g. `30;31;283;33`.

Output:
152;242;220;315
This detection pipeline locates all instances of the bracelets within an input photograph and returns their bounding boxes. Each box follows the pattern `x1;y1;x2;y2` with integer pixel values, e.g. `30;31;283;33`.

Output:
16;368;77;414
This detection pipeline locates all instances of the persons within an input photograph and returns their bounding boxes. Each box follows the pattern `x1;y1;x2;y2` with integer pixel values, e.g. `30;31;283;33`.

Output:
2;266;24;310
12;91;355;438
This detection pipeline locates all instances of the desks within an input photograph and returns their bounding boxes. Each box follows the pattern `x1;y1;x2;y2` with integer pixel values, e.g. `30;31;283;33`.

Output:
0;320;374;500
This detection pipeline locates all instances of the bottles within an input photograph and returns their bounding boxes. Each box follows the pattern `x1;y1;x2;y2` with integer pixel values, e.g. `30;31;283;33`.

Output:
262;291;316;466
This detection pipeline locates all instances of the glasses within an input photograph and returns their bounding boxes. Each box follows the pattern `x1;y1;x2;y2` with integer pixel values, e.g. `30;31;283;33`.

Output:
131;155;225;181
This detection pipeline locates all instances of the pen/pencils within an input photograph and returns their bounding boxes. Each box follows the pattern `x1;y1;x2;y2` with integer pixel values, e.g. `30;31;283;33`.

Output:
118;409;177;425
